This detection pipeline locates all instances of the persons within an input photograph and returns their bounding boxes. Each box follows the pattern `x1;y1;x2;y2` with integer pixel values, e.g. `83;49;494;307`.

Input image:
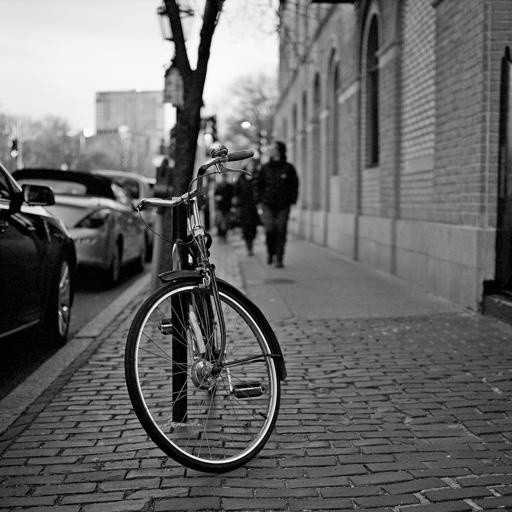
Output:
235;156;259;255
256;141;299;267
213;172;236;238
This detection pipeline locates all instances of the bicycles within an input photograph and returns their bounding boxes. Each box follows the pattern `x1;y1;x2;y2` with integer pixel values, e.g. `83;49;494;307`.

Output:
124;143;287;474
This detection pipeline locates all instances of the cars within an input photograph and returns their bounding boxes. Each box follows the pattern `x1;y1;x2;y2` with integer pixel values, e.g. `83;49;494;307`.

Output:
0;163;157;345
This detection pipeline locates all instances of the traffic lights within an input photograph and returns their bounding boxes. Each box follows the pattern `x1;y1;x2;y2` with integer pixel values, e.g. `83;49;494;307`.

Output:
9;139;20;158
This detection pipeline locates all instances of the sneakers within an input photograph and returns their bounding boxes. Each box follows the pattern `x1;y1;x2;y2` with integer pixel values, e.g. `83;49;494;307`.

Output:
267;256;284;268
